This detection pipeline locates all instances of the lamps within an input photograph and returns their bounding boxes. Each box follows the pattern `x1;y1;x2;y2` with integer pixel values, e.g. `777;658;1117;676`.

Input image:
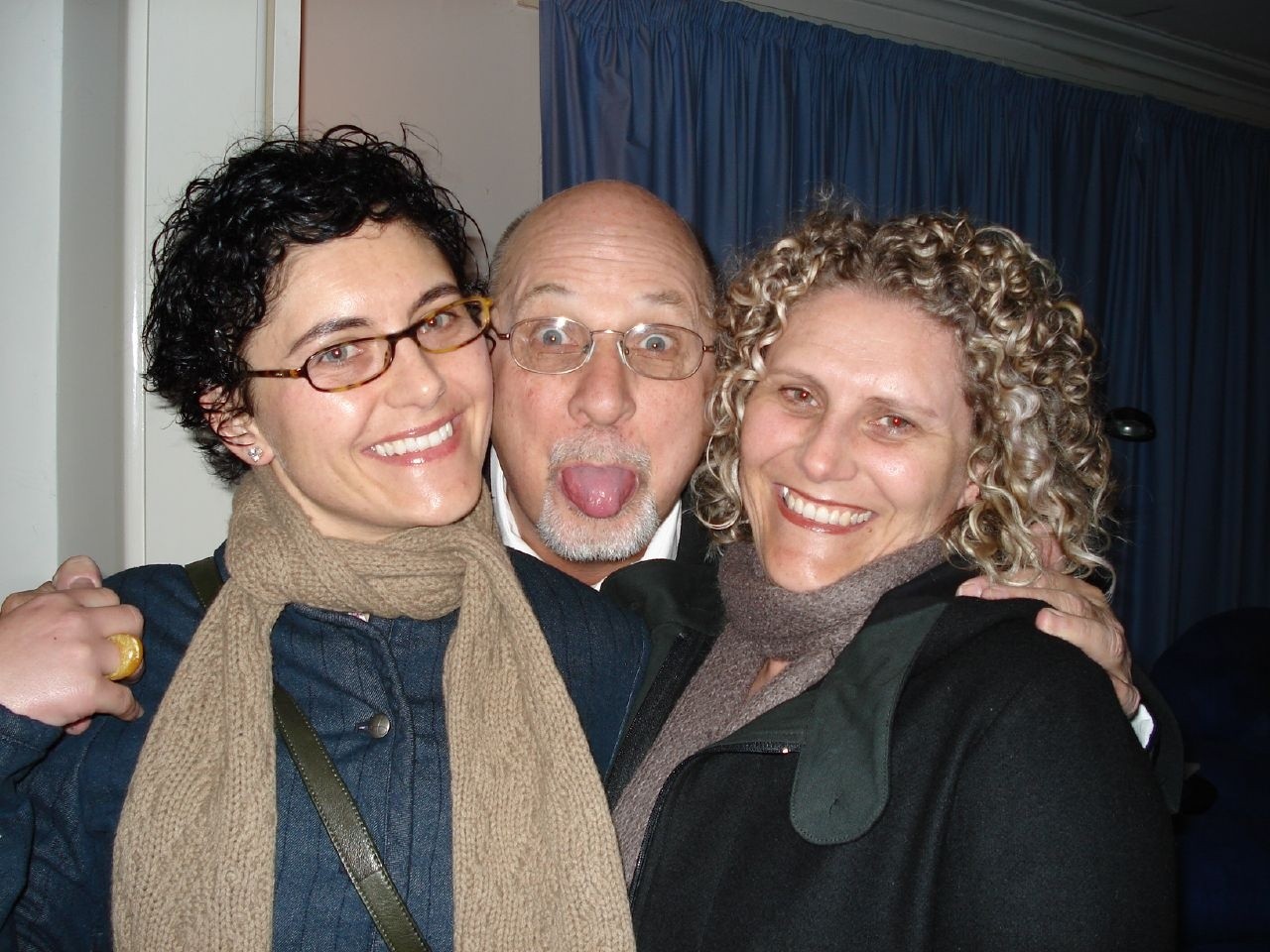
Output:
1107;406;1156;444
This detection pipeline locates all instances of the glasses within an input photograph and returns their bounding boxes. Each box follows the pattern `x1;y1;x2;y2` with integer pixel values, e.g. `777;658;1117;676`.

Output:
487;320;718;381
232;296;495;393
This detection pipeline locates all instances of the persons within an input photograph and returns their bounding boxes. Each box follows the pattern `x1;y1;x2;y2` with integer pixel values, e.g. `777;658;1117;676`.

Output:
0;175;1186;952
0;119;652;952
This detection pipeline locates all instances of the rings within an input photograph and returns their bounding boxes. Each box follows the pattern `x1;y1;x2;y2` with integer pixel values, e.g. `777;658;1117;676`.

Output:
101;633;143;681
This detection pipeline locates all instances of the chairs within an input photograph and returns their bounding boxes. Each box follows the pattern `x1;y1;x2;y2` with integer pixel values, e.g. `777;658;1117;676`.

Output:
1145;605;1270;952
